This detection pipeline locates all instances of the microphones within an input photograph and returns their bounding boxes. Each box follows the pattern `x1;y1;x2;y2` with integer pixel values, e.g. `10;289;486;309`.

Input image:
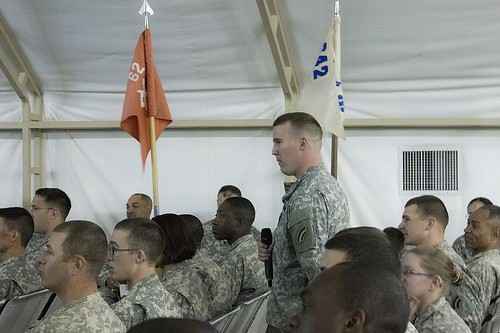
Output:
261;228;273;287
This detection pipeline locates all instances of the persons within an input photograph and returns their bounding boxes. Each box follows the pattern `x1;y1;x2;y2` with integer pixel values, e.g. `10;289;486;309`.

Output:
106;218;183;330
0;207;45;311
399;195;486;333
96;192;153;306
150;213;216;325
210;196;268;309
178;214;232;317
289;261;409;333
257;112;350;333
400;246;473;333
451;197;494;261
199;185;261;263
24;187;72;272
319;226;419;333
25;220;126;333
463;205;499;326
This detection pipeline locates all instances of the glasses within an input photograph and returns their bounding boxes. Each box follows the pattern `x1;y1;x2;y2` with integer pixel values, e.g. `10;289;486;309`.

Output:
27;205;58;215
403;269;436;278
106;245;142;256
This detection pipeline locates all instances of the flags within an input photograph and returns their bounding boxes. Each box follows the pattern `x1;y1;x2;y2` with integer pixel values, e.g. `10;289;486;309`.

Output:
296;15;347;141
119;28;173;172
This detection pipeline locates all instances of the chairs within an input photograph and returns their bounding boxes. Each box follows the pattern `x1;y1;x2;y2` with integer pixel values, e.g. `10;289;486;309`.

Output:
207;303;244;333
229;290;271;333
0;289;53;333
